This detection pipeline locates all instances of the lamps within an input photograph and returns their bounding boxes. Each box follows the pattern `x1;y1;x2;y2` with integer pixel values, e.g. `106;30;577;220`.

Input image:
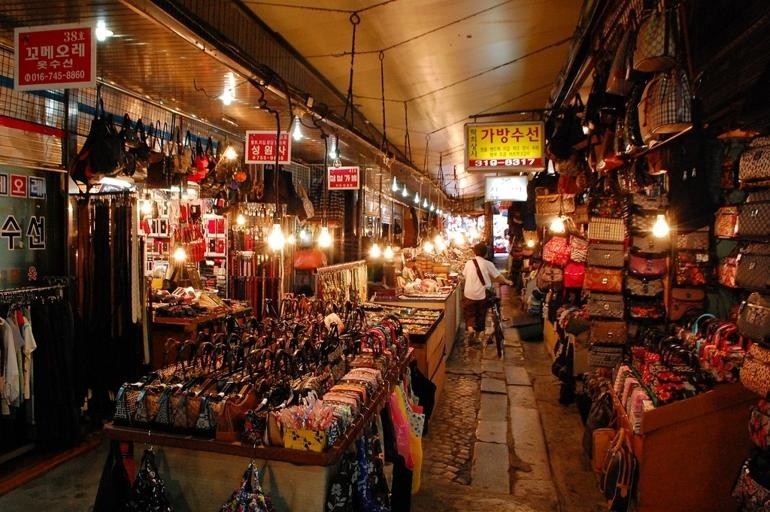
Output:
310;113;335;250
550;188;567;234
364;158;480;261
651;188;672;239
244;75;287;252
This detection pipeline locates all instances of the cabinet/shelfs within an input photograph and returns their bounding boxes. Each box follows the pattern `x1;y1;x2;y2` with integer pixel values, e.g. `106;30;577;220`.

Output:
101;275;462;512
584;365;761;512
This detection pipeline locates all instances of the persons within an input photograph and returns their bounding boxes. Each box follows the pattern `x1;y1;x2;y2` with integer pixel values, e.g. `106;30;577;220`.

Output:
462;242;513;346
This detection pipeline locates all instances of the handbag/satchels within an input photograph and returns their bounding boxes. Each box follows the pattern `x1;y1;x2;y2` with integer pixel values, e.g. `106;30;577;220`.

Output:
486;288;498;305
68;82;315;222
507;0;753;433
730;454;770;512
734;396;770;451
292;241;319;270
734;290;770;343
734;192;769;243
738;339;769;398
597;427;638;512
514;116;530;122
591;426;616;474
735;244;770;291
113;292;439;512
738;133;770;192
93;435;132;512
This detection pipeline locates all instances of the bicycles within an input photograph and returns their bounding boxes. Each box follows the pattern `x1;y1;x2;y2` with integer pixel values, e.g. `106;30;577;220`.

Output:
483;281;511;361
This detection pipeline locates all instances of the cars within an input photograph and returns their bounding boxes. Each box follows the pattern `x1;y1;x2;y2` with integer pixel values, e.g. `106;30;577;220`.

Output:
475;213;510;253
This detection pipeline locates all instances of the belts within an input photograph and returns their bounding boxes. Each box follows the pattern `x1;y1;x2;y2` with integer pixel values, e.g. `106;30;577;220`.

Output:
68;189;287;365
